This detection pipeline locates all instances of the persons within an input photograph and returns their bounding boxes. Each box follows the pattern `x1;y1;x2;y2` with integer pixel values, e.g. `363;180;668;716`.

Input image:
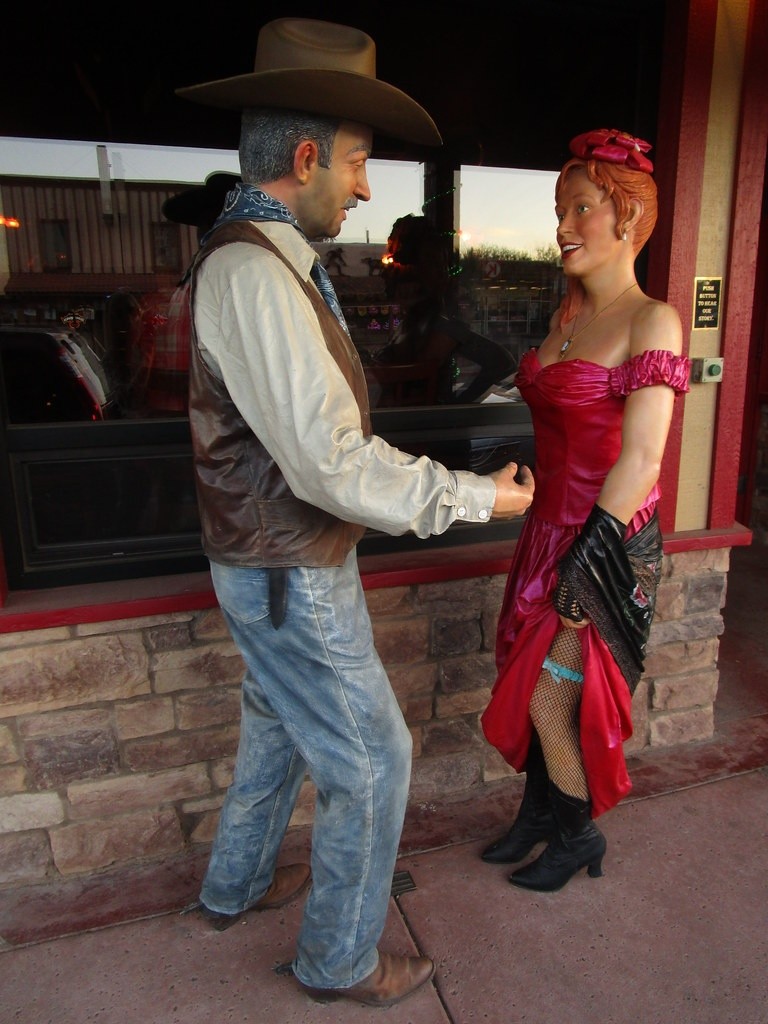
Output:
163;173;242;238
480;129;692;892
116;240;193;535
179;18;535;1005
368;216;516;553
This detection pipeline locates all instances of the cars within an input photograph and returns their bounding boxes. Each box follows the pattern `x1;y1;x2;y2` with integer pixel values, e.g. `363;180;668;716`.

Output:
0;316;125;426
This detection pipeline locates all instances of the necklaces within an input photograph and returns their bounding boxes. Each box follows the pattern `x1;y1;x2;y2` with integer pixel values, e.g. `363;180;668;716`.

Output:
558;283;637;360
